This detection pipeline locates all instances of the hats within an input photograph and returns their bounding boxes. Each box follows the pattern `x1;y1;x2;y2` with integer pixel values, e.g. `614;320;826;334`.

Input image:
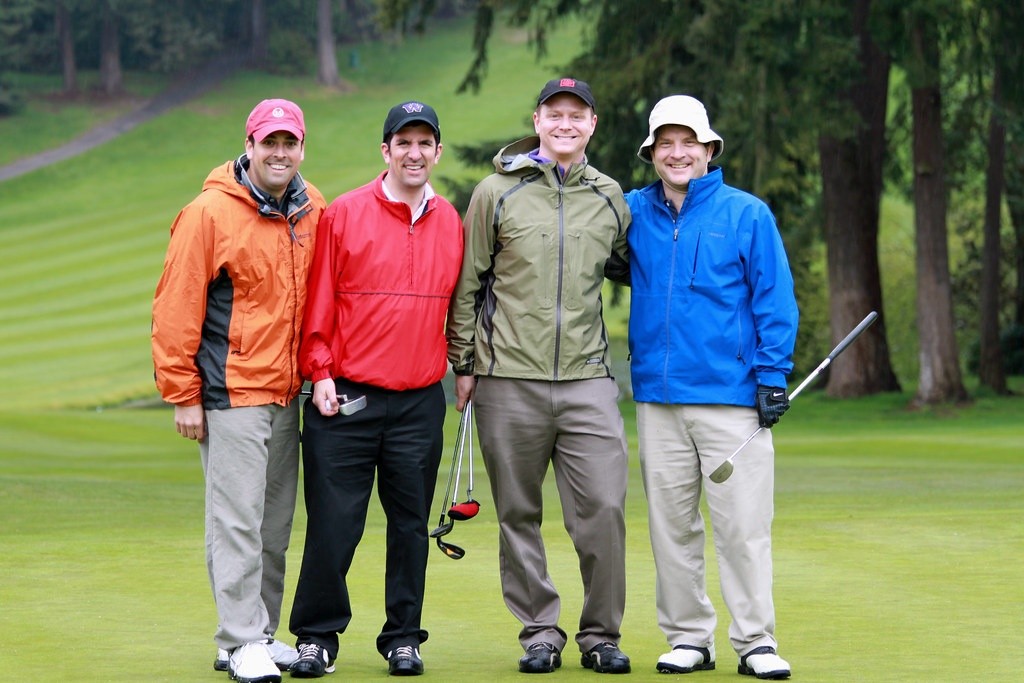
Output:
537;77;596;112
246;99;306;143
383;100;441;144
637;95;724;164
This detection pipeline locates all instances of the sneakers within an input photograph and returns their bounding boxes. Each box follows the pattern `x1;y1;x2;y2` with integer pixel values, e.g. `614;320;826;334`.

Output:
290;642;336;678
518;642;562;673
214;636;299;683
580;641;631;674
388;646;424;675
656;642;716;674
738;646;792;679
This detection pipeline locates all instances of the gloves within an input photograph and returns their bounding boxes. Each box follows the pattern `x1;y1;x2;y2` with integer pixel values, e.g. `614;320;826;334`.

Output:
756;385;791;429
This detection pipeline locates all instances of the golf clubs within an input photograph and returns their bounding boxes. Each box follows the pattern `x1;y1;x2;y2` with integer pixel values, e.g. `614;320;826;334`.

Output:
431;400;481;558
710;311;879;482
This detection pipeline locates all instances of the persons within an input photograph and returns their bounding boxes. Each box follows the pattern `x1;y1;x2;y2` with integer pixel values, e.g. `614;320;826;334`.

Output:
620;94;799;678
289;102;465;677
151;99;328;680
446;79;632;675
